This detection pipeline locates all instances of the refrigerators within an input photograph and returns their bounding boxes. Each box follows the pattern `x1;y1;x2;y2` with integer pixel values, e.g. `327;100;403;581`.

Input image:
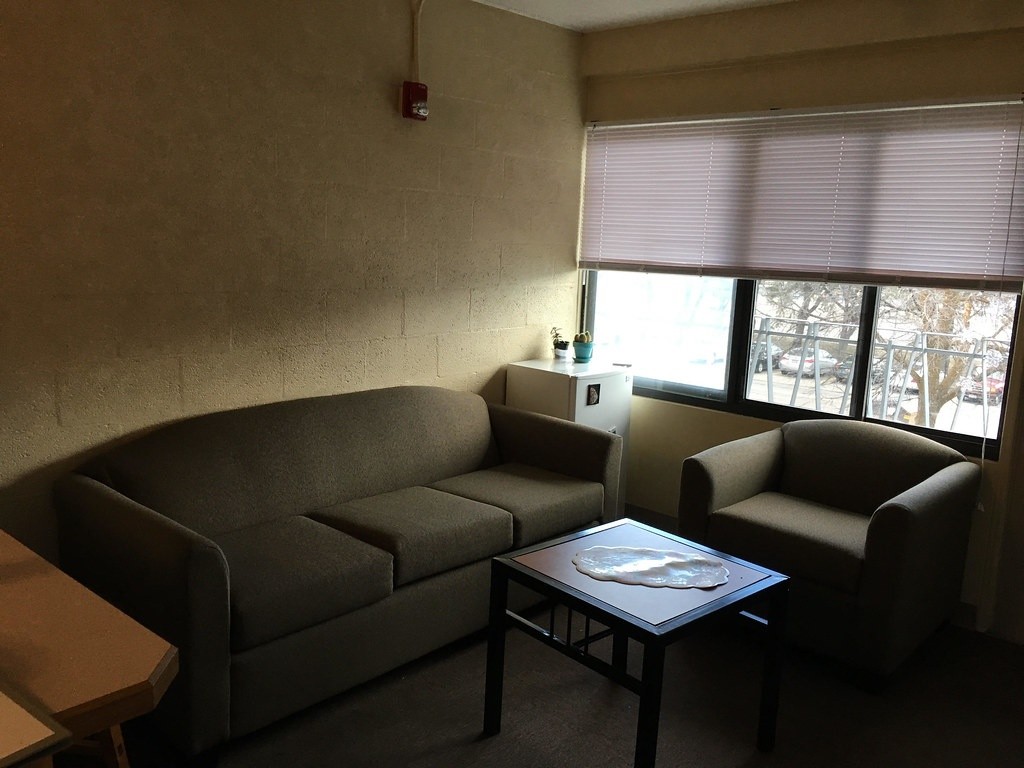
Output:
507;358;634;520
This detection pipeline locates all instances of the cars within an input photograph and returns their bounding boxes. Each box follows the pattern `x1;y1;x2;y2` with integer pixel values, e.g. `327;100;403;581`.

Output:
780;348;837;378
891;363;945;393
963;366;1005;405
752;342;783;373
834;355;880;384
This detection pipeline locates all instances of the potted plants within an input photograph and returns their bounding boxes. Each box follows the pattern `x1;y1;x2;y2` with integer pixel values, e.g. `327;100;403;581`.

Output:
573;331;596;359
551;326;570;357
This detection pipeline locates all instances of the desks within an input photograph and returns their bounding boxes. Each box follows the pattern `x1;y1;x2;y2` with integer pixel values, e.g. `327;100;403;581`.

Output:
477;517;795;768
0;529;181;768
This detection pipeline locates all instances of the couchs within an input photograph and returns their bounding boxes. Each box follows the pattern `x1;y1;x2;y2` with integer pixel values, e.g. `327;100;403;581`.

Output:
677;418;981;699
52;385;625;768
